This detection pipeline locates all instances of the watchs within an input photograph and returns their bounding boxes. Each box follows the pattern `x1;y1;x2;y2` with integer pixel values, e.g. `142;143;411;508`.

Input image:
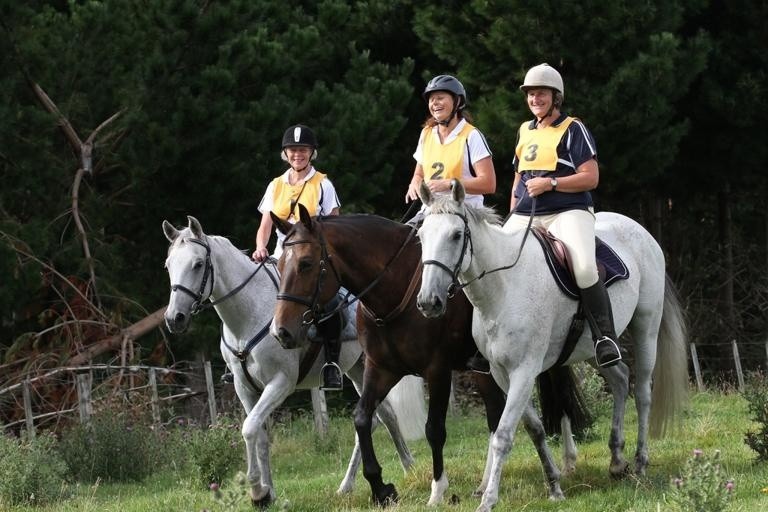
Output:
550;176;558;192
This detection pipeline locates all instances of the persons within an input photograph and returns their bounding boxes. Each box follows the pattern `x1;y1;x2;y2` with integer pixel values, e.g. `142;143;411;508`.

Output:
405;74;496;224
251;123;344;388
503;62;622;368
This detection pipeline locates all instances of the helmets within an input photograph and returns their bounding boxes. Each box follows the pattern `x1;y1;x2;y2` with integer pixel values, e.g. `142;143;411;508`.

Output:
281;125;318;150
519;63;564;102
422;74;467;110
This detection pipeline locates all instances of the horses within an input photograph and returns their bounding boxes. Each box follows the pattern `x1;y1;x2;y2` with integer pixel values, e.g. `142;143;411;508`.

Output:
415;177;691;512
267;201;595;511
163;213;429;512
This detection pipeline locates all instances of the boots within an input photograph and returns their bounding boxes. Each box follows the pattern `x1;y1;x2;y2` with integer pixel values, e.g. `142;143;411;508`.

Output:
320;299;342;388
577;278;619;368
471;357;490;372
